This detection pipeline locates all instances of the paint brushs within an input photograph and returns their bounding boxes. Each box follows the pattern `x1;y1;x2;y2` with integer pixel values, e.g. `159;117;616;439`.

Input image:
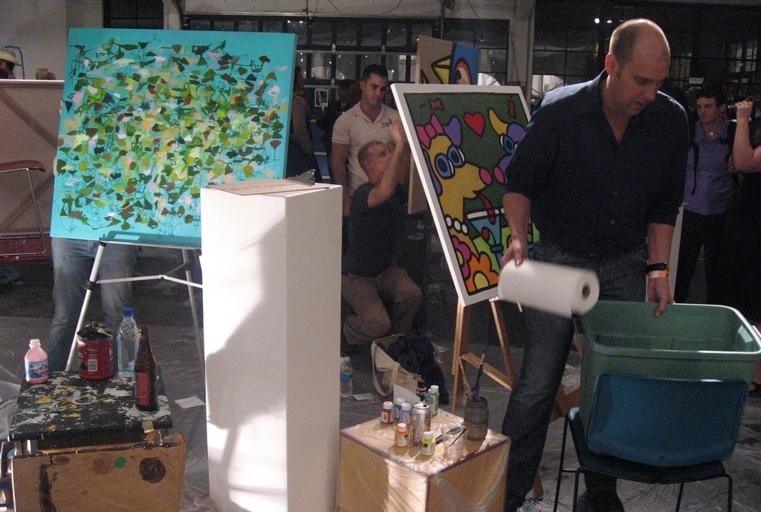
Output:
449;429;465;447
457;349;485;402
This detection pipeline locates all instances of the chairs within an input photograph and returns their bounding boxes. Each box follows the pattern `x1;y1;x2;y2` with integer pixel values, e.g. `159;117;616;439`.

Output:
553;372;748;512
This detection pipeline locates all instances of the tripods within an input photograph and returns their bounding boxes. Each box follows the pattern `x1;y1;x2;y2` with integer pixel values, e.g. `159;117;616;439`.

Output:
64;241;204;373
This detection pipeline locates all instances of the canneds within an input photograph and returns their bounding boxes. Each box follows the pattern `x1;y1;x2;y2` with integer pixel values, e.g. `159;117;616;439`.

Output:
412;403;431;446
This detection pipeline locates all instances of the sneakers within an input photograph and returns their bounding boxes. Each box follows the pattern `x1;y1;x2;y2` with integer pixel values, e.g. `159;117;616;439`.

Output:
577;488;624;512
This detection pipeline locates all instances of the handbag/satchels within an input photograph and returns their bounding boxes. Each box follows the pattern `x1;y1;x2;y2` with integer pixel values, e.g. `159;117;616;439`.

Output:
371;331;448;405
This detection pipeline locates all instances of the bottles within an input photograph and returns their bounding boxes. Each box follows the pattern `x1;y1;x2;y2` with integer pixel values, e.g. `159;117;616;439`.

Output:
380;380;440;456
342;356;352;398
115;307;140;379
133;326;158;412
24;339;49;385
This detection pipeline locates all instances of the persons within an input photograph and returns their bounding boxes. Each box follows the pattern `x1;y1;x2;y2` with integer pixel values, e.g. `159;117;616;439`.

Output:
332;64;407;218
674;84;740;303
0;46;24;79
716;97;760;325
45;238;136;371
501;18;690;512
341;119;423;355
286;67;314;175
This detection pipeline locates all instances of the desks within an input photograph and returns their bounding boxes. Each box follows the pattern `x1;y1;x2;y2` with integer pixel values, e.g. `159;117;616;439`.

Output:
338;406;511;512
8;359;172;459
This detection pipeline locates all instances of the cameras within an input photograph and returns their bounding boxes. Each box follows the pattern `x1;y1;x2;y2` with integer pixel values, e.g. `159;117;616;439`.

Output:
726;101;755;119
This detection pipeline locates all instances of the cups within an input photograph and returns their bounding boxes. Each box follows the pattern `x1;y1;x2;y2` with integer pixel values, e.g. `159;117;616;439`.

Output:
465;398;488;440
441;425;465;462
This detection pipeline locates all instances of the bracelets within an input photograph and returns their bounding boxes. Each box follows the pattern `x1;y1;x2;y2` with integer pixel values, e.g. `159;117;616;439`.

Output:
643;262;668;272
646;270;668;280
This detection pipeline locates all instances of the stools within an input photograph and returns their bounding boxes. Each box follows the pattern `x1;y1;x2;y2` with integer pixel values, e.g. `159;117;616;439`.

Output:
0;159;49;257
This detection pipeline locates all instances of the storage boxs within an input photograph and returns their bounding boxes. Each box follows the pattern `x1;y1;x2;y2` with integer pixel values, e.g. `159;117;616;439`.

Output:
581;300;761;446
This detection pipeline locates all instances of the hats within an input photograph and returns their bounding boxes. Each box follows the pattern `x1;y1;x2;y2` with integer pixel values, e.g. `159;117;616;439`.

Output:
0;48;22;65
